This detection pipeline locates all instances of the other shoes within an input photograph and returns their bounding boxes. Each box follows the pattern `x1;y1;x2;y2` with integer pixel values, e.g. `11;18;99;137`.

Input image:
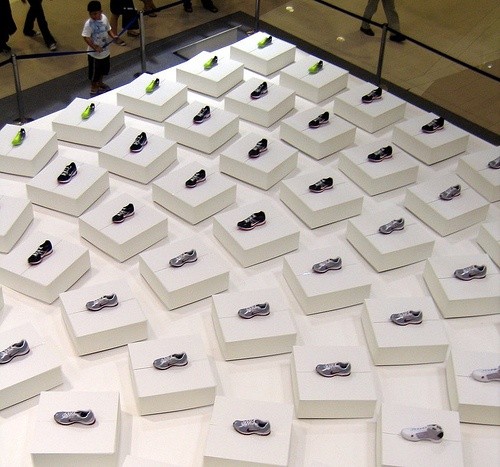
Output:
30;30;40;37
361;26;375;36
203;4;218;13
390;34;405;41
98;83;109;89
184;5;192;12
128;32;140;37
90;89;97;96
116;38;126;45
49;44;56;49
1;44;11;52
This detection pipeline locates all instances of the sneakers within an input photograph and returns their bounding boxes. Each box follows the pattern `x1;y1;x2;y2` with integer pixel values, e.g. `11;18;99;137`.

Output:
361;87;382;102
193;106;211;123
308;60;323;73
54;409;95;426
146;79;159;93
0;129;500;383
251;82;268;99
204;56;218;69
233;419;271;435
401;423;443;443
81;104;95;118
258;36;272;47
422;118;445;133
309;111;329;128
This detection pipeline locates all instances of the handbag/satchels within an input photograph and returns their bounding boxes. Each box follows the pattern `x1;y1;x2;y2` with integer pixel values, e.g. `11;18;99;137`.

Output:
122;0;139;30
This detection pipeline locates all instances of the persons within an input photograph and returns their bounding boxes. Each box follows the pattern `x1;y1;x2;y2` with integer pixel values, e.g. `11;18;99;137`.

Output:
360;0;406;43
0;0;17;53
109;0;139;46
183;0;219;14
81;0;119;96
20;0;57;51
141;0;160;17
